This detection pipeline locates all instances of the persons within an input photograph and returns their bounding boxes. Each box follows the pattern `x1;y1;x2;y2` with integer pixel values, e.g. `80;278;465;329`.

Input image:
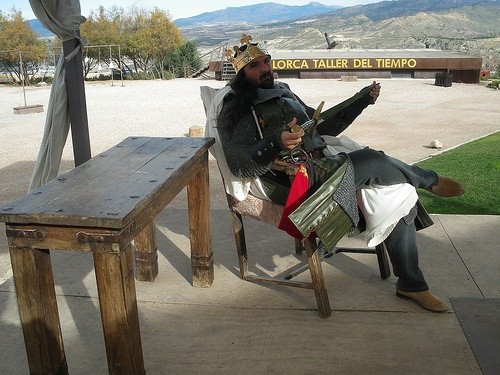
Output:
216;33;465;312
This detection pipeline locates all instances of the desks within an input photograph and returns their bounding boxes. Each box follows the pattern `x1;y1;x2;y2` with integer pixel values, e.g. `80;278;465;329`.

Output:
0;137;215;375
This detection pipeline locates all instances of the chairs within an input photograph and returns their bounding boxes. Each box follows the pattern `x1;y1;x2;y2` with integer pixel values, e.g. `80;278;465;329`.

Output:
199;86;391;320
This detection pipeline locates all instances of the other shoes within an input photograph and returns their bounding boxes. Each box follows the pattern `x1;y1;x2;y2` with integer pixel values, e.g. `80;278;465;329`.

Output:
395;289;451;313
424;175;465;198
